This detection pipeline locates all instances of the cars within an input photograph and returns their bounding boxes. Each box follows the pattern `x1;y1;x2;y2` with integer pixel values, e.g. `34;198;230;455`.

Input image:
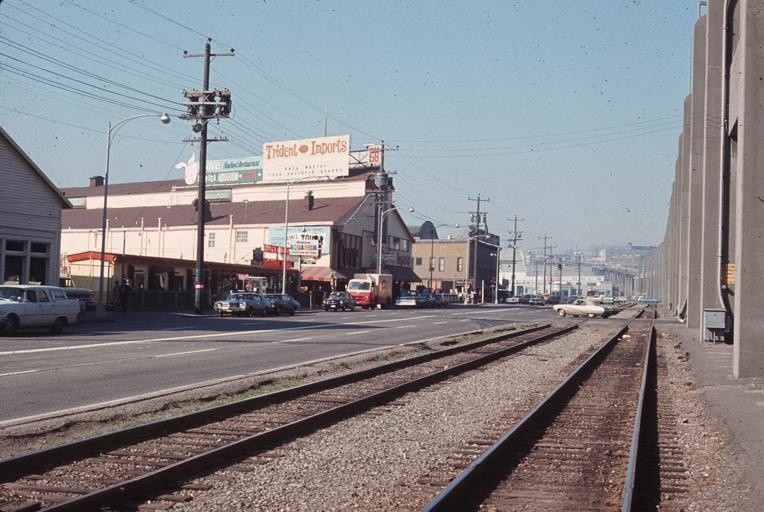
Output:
321;291;355;312
428;294;449;308
551;297;621;319
59;277;94;311
259;292;301;316
0;283;81;336
506;291;651;304
210;291;272;318
394;292;428;308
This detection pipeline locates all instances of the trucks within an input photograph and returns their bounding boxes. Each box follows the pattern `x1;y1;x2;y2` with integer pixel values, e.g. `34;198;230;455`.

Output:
344;273;394;309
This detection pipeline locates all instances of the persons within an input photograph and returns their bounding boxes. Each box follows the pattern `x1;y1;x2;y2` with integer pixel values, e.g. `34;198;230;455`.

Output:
118;279;129;312
111;280;121;311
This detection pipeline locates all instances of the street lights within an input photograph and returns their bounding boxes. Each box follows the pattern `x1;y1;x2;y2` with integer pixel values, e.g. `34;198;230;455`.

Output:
280;174;333;294
527;248;537;294
493;242;517;305
93;112;170;323
573;250;583;294
377;205;414;274
464;234;490;303
430;222;460;289
